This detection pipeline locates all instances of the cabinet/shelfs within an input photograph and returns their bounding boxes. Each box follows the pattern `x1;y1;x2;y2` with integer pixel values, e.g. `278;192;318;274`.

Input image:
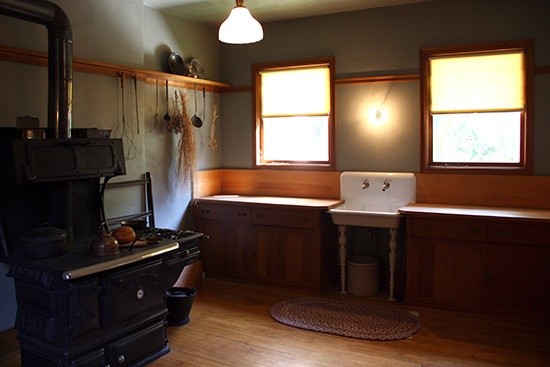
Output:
197;199;337;297
4;239;180;367
0;45;231;93
399;210;550;324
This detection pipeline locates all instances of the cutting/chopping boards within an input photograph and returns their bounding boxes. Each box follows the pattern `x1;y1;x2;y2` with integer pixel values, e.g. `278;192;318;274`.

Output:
121;236;162;247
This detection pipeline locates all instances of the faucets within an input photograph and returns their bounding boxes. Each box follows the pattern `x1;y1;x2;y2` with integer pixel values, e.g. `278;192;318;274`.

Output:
361;178;371;191
381;177;393;193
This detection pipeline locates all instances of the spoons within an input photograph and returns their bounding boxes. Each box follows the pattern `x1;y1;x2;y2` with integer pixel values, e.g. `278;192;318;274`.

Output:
192;83;203;128
164;78;170;121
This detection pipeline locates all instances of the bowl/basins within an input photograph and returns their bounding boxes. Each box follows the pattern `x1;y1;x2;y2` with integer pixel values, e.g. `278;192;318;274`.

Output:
121;220;147;231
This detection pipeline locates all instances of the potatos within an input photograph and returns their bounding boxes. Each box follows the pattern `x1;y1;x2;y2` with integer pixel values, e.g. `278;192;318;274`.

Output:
114;226;135;243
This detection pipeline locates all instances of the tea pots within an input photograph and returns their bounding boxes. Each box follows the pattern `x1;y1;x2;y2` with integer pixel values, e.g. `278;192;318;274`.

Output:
90;220;120;256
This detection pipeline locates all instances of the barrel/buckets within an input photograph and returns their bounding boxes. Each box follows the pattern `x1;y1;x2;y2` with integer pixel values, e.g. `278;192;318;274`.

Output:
347;255;381;297
165;287;197;327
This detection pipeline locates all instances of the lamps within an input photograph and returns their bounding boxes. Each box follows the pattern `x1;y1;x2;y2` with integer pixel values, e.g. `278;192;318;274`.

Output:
217;0;264;45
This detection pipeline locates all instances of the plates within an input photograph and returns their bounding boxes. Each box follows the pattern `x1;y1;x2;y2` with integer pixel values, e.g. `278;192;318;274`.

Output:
168;52;184;75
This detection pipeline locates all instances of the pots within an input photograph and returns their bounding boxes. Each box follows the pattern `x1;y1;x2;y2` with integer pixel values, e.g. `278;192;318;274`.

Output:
21;222;67;259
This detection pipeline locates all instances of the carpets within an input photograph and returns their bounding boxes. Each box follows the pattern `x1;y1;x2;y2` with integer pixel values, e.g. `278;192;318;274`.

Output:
270;297;422;341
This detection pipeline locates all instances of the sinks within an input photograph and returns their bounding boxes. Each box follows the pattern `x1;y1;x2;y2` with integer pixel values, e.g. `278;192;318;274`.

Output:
327;172;417;228
